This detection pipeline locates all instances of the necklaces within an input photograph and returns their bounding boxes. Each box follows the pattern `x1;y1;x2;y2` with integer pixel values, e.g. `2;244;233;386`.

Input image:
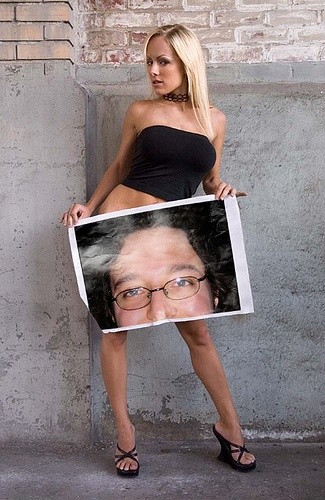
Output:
163;93;189;102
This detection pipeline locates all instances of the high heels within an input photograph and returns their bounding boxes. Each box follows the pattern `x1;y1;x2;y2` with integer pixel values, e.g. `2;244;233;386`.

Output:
212;424;257;471
114;441;140;477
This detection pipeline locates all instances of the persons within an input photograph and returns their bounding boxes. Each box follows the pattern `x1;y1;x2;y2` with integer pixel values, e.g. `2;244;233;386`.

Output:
59;24;256;476
74;198;241;330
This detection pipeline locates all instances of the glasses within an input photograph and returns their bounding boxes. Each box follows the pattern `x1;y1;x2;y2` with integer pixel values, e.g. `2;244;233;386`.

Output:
112;273;207;311
